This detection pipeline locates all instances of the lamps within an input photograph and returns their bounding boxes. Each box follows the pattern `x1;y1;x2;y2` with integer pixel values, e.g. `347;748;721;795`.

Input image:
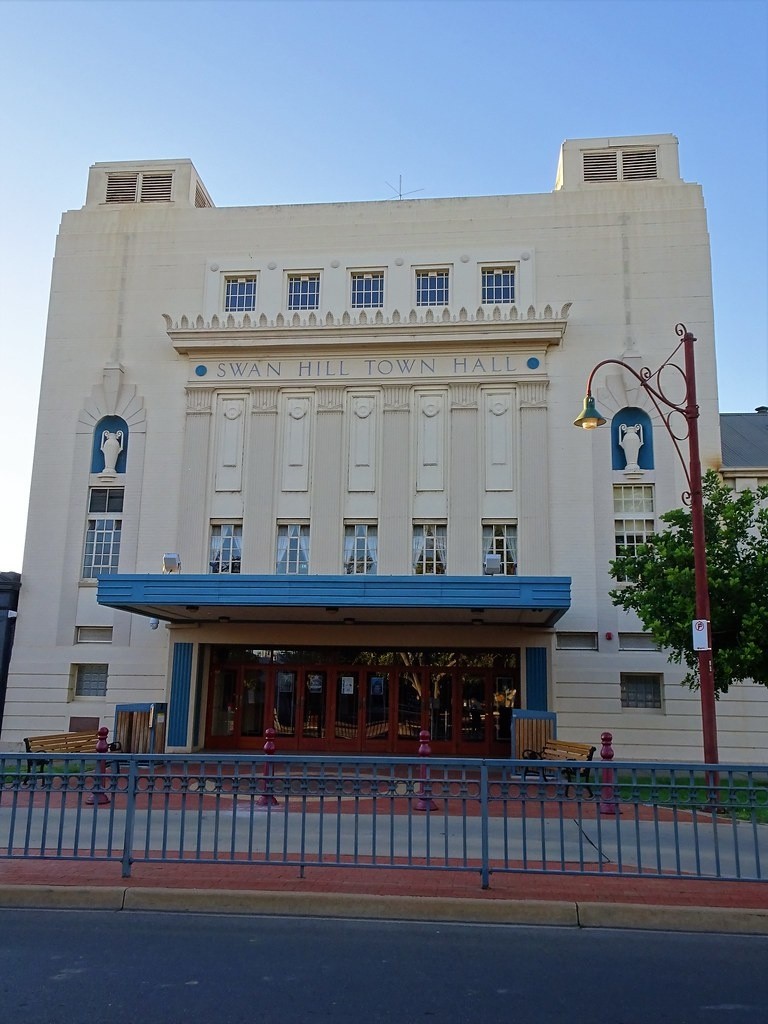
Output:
472;619;483;625
483;554;500;576
471;609;484;615
218;616;231;622
150;618;159;630
186;605;199;613
326;608;339;615
162;553;181;575
343;618;356;624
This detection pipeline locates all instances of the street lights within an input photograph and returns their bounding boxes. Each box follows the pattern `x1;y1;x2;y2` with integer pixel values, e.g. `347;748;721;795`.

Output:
572;323;726;814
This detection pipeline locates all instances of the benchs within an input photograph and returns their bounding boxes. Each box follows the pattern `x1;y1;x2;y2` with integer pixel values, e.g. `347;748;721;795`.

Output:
22;730;123;788
521;738;596;798
